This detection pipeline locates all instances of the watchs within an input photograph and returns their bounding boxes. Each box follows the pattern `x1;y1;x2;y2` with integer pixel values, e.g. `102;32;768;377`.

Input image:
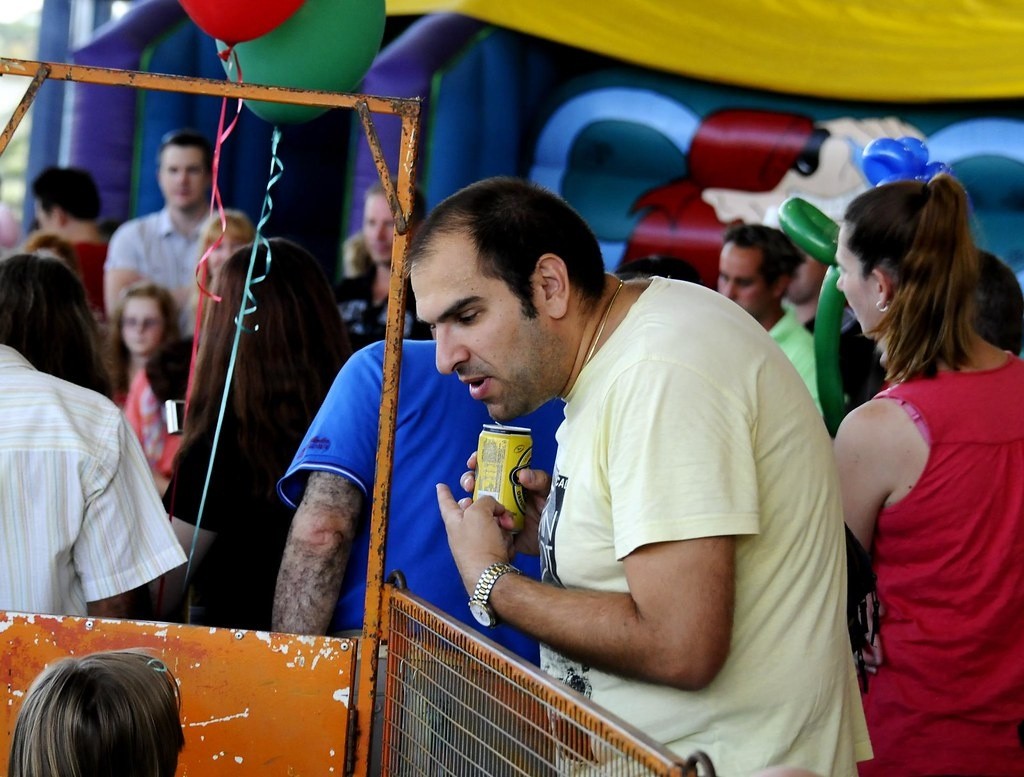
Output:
465;561;523;628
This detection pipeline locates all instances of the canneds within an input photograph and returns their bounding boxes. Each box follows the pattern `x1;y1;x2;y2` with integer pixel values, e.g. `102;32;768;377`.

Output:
473;422;534;534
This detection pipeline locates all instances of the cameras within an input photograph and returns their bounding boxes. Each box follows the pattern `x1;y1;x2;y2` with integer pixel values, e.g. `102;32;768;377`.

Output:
165;399;186;435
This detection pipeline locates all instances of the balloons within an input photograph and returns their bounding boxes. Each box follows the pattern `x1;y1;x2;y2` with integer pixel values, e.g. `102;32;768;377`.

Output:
776;137;953;442
176;0;304;63
215;0;387;125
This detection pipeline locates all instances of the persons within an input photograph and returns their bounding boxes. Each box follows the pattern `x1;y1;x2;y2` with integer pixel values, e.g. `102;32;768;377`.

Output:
0;254;112;400
829;174;1022;755
148;237;350;637
20;127;253;508
716;225;852;422
0;337;191;620
334;172;434;356
271;340;553;777
7;647;184;777
406;175;878;777
762;195;888;432
